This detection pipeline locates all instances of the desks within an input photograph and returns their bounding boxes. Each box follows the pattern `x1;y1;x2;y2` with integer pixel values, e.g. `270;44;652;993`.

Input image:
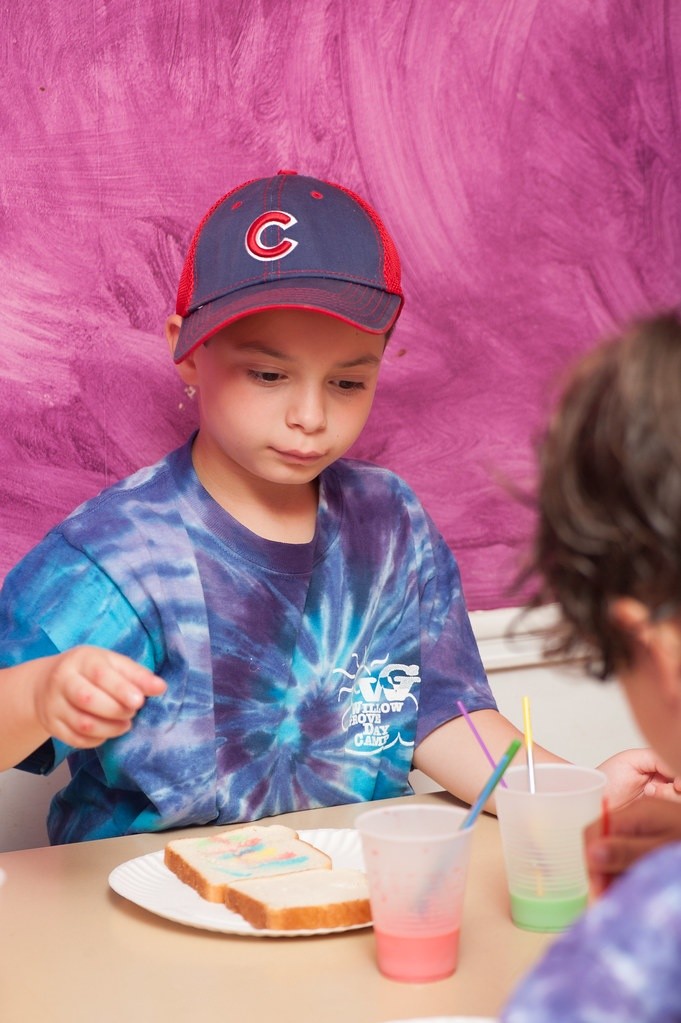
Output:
0;789;552;1023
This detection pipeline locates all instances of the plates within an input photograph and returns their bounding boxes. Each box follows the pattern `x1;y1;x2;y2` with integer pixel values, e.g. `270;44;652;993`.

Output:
106;828;386;937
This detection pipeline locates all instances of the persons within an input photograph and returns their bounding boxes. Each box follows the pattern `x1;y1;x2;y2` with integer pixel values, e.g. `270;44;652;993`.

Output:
502;309;681;1023
0;173;681;837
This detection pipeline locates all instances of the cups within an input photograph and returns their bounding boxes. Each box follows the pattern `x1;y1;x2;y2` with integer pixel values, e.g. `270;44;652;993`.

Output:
357;804;474;982
491;763;612;935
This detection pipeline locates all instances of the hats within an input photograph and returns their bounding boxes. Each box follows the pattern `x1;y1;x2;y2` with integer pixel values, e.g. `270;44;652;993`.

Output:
173;170;405;364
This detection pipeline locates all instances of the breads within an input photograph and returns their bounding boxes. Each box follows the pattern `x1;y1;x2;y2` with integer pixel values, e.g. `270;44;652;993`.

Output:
222;867;371;931
163;824;333;904
